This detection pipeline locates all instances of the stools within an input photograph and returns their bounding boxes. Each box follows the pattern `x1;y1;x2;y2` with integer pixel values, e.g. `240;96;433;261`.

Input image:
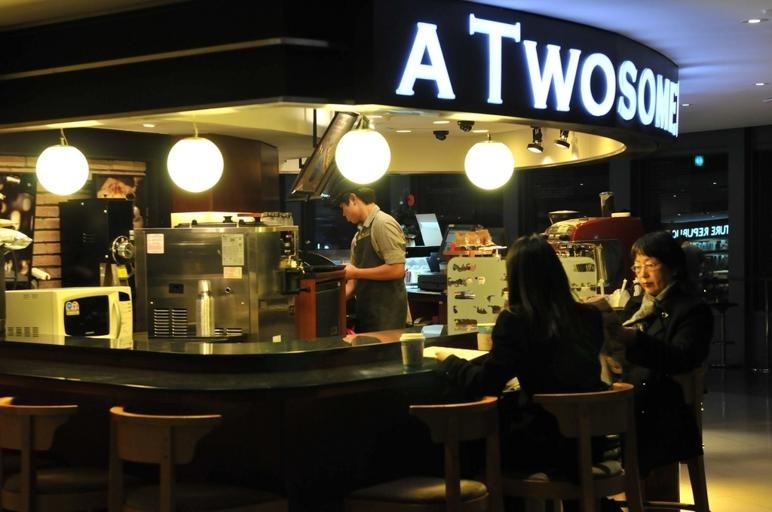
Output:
708;300;738;369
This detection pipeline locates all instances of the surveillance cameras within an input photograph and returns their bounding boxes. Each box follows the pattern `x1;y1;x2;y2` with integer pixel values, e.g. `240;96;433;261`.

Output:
456;121;475;133
433;131;448;141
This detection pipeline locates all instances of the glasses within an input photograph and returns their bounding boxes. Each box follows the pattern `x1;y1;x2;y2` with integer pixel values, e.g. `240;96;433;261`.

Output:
631;263;661;273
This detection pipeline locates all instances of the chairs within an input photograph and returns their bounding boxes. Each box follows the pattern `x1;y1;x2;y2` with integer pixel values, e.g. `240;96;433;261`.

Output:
507;382;644;511
0;395;79;512
344;394;506;511
604;368;709;512
107;402;223;512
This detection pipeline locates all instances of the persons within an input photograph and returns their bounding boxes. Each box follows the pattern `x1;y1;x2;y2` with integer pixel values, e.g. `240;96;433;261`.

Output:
336;179;408;333
605;229;715;477
679;235;706;275
437;234;605;480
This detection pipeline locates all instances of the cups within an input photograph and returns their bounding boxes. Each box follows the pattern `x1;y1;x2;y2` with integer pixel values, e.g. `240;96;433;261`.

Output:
599;191;615;217
195;279;216;338
477;322;496;351
406;272;411;282
399;333;426;370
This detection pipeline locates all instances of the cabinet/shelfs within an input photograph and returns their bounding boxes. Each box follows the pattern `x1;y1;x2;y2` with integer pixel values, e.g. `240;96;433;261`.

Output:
690;235;729;301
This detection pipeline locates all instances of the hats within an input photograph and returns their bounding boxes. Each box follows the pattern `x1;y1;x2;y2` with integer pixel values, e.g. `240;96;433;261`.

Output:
329;179;371;206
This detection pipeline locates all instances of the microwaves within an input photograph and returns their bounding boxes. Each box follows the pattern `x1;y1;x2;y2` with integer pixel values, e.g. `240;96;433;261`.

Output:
4;286;134;351
